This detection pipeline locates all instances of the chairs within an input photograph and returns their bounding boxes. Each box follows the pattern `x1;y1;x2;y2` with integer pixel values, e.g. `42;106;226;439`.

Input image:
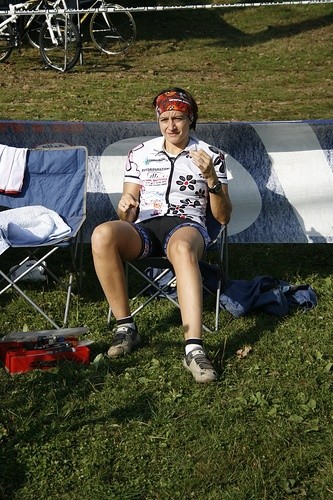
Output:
0;144;89;332
106;215;228;335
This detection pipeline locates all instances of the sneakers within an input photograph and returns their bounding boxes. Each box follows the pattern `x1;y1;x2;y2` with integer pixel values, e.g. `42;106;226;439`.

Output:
108;327;140;357
183;349;217;383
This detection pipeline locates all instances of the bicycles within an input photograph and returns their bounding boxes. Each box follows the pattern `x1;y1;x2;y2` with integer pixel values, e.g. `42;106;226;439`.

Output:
23;0;137;55
0;0;82;72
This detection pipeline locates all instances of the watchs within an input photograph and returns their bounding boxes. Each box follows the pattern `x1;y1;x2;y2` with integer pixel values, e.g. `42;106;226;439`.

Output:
208;181;222;193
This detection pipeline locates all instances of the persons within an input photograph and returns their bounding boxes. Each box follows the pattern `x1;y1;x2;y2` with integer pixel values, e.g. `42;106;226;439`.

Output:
90;87;232;383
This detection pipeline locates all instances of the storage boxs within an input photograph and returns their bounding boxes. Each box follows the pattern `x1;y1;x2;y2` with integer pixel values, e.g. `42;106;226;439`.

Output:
0;337;90;375
